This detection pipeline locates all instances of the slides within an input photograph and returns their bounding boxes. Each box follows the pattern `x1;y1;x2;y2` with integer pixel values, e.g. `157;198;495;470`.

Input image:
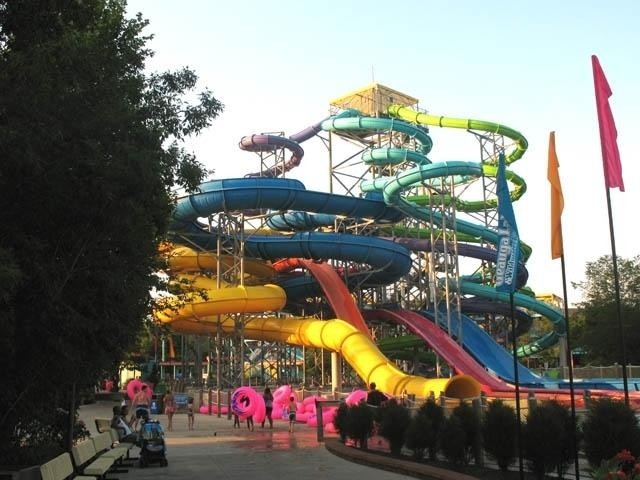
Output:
378;305;640;413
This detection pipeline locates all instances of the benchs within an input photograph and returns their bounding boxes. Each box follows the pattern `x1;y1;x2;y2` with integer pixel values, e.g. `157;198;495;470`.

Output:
40;418;140;480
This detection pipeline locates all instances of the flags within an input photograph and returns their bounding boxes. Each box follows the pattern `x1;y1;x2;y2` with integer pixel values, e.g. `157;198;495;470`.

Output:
591;54;625;193
547;131;564;259
494;153;521;293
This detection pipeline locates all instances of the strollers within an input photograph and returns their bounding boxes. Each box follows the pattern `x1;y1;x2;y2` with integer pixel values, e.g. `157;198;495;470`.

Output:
138;421;168;467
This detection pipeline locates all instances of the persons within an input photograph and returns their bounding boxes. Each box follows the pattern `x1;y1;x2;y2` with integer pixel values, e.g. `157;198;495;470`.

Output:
95;372;389;455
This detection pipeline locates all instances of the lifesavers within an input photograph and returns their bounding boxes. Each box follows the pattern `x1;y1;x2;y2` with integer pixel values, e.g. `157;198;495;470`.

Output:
127;380;152;401
199;386;391;433
